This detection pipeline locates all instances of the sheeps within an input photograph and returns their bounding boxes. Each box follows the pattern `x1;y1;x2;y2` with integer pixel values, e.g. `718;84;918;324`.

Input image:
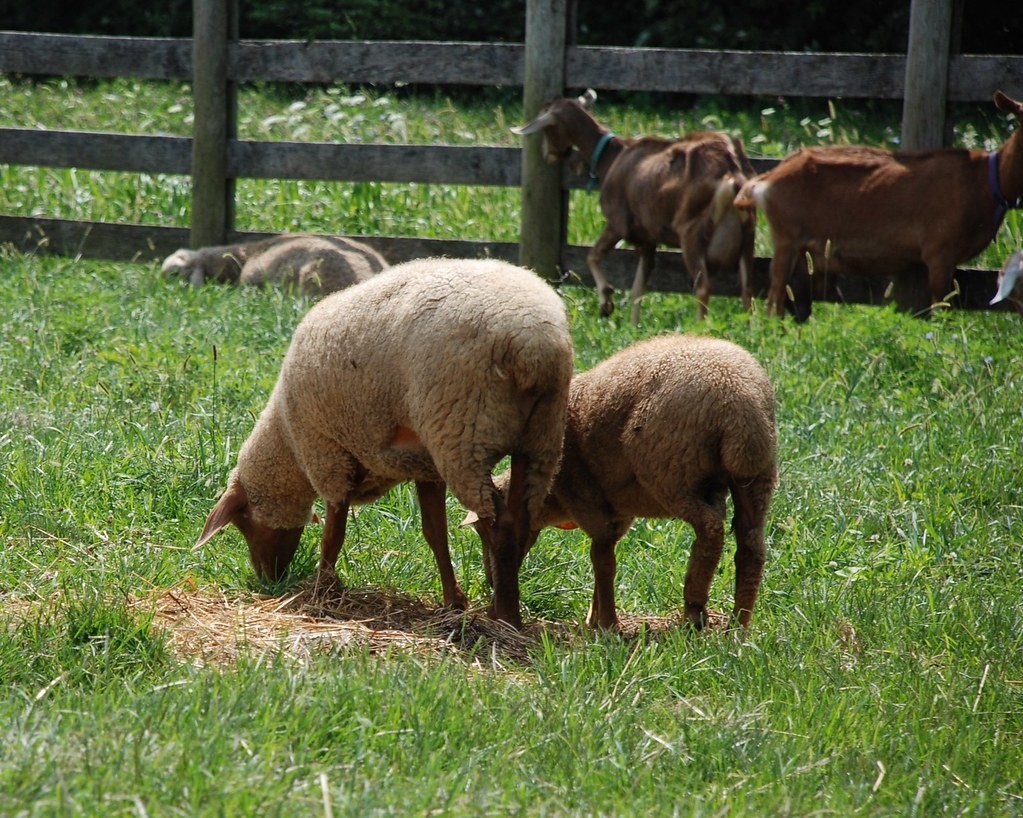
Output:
459;334;779;631
511;88;758;329
161;234;389;293
188;256;571;626
732;91;1023;322
